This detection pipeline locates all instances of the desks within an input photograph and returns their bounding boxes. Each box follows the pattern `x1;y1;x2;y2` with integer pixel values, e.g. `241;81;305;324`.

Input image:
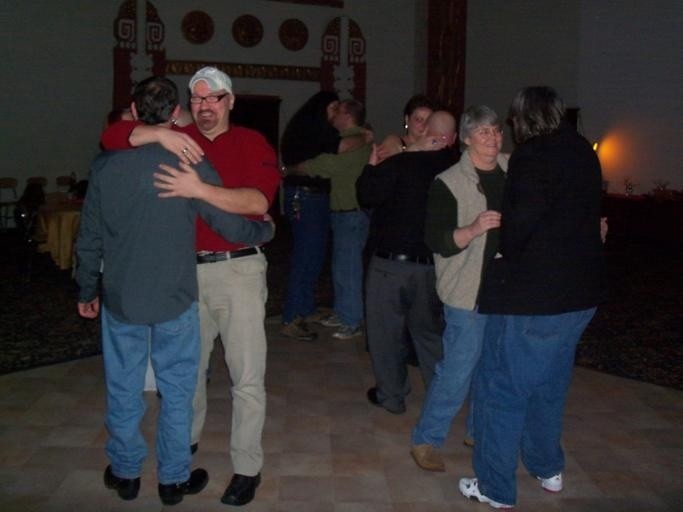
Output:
36;190;84;269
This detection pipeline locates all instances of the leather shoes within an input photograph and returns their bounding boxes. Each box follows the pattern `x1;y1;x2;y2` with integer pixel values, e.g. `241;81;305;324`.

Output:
104;464;142;500
159;467;208;504
222;471;261;505
367;386;384;407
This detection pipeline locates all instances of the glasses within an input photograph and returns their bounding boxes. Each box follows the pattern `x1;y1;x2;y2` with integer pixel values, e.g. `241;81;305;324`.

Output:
479;128;504;138
190;93;226;104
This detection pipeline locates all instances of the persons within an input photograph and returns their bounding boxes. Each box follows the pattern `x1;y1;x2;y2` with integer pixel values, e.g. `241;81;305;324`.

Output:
277;85;462;416
70;73;274;506
408;85;614;510
100;65;280;508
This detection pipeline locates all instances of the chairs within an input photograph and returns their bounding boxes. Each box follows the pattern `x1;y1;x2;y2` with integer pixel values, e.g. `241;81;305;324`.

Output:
0;177;20;228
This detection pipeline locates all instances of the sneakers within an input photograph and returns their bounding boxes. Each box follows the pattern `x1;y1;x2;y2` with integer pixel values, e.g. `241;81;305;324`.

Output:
412;442;446;472
278;320;318;343
459;477;515;508
537;471;563;493
320;313;363;340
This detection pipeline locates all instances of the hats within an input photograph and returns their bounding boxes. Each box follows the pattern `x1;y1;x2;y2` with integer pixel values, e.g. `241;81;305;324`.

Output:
188;66;232;94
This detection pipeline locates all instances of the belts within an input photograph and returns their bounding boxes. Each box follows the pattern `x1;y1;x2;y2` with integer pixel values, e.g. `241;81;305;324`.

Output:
197;245;264;265
373;248;436;264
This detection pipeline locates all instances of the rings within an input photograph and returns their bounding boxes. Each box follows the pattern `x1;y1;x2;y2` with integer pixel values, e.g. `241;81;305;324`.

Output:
181;148;189;154
